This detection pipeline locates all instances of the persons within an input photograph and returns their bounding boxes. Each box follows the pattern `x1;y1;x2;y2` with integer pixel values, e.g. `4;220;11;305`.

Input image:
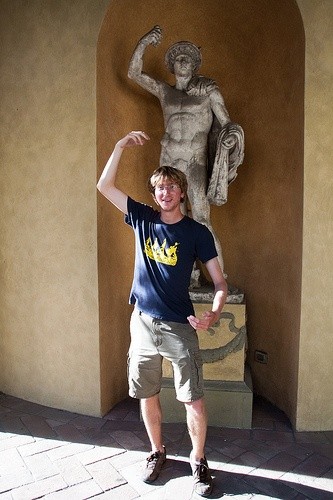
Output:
96;131;226;499
128;25;245;293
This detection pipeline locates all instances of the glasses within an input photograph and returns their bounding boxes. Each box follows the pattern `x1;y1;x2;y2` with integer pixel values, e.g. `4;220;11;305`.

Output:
152;184;180;190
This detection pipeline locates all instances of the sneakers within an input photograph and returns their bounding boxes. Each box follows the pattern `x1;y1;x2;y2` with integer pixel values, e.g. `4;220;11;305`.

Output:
189;452;213;497
142;444;167;484
